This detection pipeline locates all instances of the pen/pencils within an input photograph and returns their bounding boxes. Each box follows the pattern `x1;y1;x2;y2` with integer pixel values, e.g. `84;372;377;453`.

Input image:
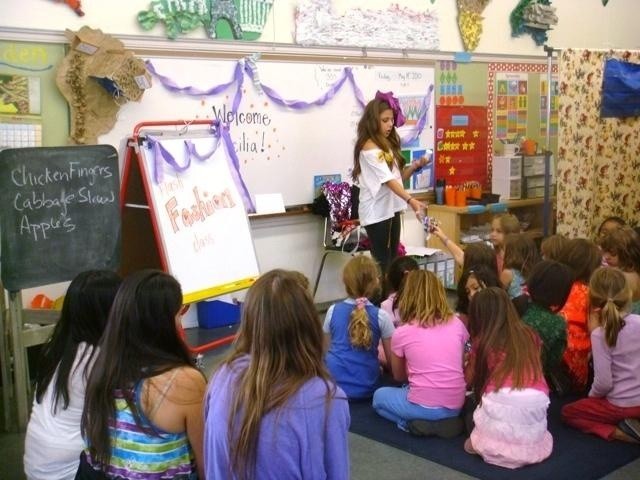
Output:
437;176;481;192
500;133;519;144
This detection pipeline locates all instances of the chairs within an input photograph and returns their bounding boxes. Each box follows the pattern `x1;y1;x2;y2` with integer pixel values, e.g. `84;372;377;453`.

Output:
310;185;377;301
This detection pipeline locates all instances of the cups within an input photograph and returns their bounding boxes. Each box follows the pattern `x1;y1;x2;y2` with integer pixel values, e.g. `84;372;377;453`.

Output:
446;186;482;207
500;140;538;157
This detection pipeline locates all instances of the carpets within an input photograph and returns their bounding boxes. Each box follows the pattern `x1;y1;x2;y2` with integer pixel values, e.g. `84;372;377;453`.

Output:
344;400;640;480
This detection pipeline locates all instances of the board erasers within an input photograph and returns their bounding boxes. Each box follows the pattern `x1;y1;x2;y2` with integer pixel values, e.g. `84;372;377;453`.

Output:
286;206;304;211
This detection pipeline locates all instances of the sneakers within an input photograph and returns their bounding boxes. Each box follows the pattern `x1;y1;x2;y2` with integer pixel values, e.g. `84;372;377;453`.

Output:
406;418;466;441
616;416;640;440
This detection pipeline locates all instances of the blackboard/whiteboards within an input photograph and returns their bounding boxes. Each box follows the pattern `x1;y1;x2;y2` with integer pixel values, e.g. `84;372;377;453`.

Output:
134;119;262;305
83;48;437;220
0;144;123;292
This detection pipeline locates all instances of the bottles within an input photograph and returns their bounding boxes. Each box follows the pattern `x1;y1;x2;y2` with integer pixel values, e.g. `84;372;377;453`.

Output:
434;179;445;205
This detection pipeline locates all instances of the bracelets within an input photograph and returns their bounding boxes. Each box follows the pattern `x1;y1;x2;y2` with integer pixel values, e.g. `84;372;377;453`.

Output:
407;196;415;203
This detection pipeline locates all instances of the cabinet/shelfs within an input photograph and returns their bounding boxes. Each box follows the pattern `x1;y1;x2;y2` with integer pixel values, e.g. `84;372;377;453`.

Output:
425;194;555;297
490;156;523;201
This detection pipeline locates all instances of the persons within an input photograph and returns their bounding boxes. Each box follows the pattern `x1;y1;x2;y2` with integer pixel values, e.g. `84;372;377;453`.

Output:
201;268;350;480
20;269;122;480
348;97;433;269
76;267;208;480
